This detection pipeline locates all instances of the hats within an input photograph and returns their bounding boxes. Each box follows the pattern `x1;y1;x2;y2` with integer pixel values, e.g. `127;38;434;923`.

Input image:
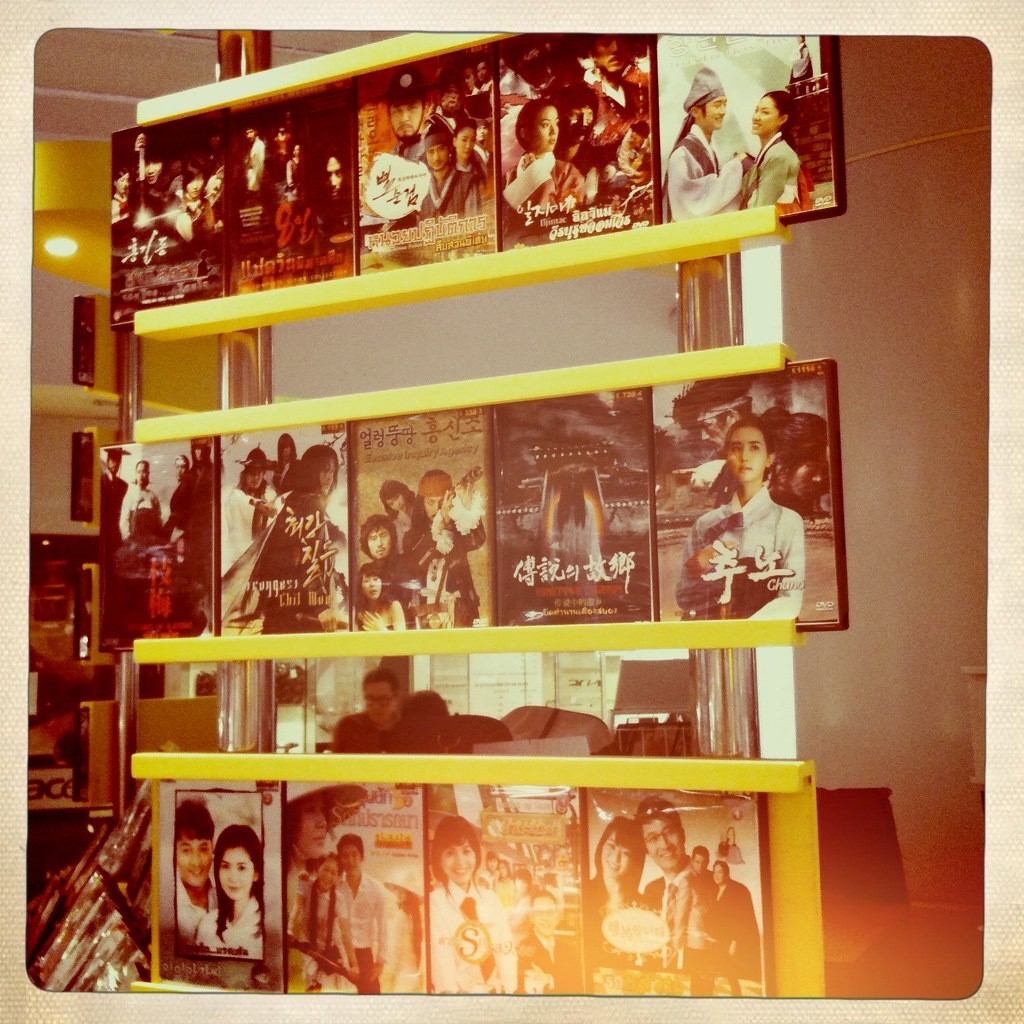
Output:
287;782;368;832
104;448;131;455
684;66;725;113
235;442;279;468
387;67;425;107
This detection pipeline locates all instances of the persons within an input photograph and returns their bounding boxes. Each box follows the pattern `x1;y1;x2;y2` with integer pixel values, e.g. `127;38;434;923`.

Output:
112;133;224;277
334;667;402;754
587;795;761;965
242;125;344;251
357;469;486;631
500;36;800;231
177;801;263;958
673;417;806;620
429;816;578;994
386;55;493;226
99;438;213;635
286;781;423;995
551;472;605;623
224;433;338;634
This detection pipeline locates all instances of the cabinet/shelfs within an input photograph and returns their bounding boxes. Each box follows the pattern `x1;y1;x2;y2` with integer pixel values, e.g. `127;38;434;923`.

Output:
126;32;830;998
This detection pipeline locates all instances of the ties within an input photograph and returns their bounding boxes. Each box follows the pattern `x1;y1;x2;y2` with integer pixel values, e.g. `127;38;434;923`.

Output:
665;885;678;933
